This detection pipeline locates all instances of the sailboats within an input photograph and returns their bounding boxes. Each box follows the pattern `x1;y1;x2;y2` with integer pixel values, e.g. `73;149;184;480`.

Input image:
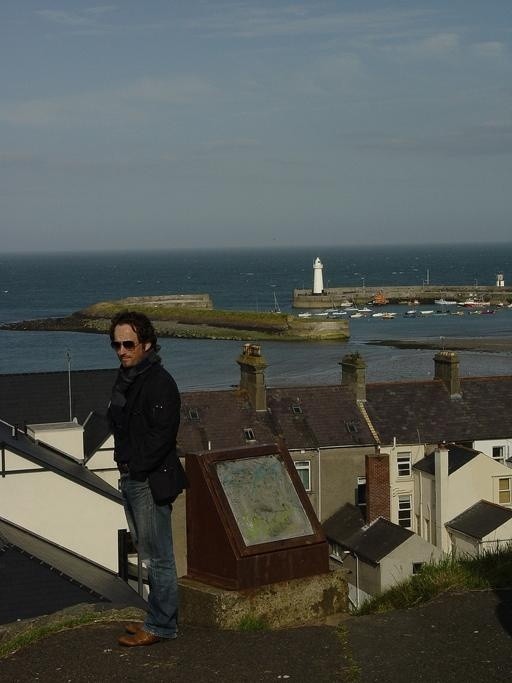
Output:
261;290;284;315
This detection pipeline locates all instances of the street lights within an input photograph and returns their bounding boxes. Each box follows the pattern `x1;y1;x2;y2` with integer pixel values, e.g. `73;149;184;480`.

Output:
361;277;365;289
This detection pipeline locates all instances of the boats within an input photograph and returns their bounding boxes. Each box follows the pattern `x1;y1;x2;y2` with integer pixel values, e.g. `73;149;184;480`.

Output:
295;292;512;320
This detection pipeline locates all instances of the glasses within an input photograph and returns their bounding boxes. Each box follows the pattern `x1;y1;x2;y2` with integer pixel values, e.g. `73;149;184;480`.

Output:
110;340;140;351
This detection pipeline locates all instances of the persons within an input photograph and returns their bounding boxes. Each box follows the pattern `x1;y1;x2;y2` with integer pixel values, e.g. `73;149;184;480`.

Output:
103;306;182;647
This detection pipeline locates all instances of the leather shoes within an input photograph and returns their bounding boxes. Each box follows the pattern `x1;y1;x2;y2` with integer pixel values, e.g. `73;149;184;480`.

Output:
118;621;160;646
